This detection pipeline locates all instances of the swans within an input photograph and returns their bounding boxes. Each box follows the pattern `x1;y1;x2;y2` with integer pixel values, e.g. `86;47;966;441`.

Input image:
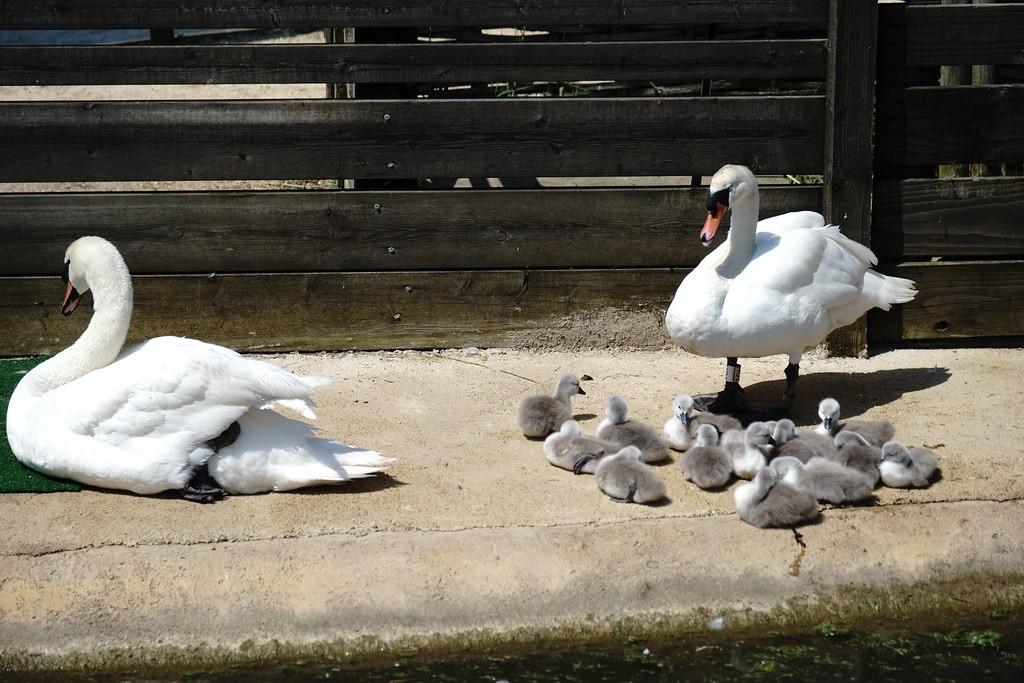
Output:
666;165;922;414
518;372;938;529
5;236;394;505
